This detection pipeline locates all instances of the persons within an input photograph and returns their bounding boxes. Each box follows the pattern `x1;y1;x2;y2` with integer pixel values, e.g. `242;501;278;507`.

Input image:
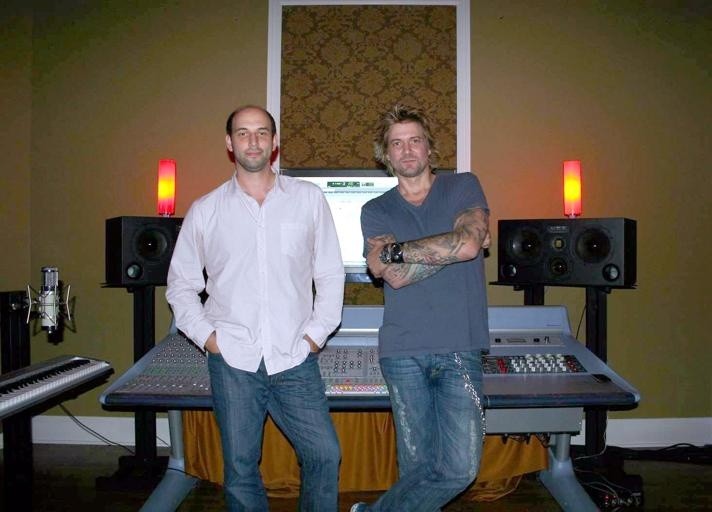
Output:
349;103;493;511
164;103;348;512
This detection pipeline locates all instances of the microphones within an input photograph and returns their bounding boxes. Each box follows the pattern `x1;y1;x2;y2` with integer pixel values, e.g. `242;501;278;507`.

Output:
40;266;58;331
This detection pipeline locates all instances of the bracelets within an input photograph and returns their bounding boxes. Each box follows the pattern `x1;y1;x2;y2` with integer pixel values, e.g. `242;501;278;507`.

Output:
379;240;405;265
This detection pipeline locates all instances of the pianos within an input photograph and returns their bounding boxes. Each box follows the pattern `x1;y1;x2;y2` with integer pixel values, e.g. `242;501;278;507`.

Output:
1;353;112;422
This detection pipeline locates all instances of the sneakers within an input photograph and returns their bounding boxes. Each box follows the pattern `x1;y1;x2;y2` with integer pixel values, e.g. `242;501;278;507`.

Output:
350;502;365;512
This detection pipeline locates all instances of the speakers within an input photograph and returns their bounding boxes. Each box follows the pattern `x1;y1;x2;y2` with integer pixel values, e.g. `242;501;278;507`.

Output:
104;216;184;284
497;217;638;286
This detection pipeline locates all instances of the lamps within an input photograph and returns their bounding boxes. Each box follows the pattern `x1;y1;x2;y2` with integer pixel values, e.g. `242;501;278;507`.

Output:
157;160;175;218
563;159;581;220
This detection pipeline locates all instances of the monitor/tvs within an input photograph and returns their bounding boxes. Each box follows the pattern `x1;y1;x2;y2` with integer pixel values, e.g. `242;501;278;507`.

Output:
267;169;457;285
